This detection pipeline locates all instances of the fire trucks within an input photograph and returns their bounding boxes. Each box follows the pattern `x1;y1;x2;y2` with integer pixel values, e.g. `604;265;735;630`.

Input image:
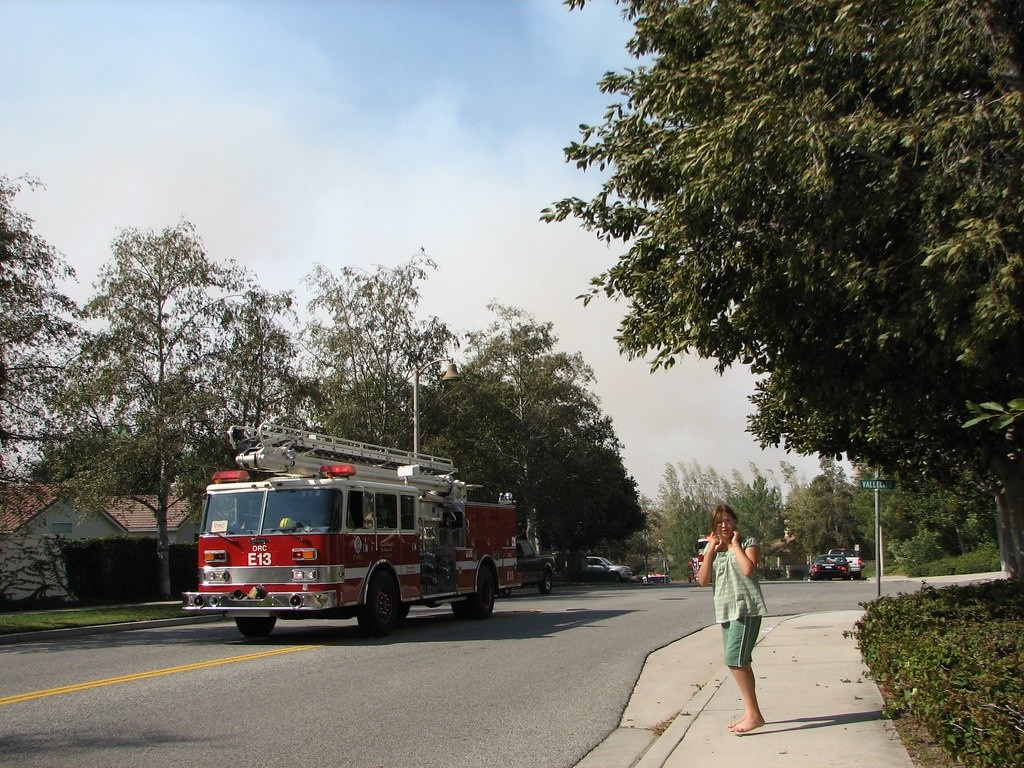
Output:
688;534;710;584
183;421;522;640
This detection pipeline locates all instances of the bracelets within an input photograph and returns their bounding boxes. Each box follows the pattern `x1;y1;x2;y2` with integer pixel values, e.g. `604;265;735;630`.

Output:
732;544;740;549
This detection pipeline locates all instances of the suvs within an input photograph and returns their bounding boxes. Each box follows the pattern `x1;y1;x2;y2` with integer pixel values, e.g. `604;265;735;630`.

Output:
564;556;632;583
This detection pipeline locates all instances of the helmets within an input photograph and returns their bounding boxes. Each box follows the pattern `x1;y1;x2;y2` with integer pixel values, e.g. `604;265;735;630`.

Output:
280;517;294;529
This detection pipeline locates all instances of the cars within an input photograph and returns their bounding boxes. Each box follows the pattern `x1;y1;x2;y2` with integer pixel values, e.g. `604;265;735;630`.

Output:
811;554;853;581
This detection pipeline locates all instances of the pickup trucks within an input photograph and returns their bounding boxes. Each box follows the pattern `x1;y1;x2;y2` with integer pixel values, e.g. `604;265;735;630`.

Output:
498;541;556;599
827;549;862;580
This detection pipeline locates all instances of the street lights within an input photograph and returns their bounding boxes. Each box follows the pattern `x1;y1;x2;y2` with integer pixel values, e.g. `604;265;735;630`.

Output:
412;356;463;459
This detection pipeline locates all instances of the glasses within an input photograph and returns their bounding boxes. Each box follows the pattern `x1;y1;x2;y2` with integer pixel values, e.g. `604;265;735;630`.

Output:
714;518;733;528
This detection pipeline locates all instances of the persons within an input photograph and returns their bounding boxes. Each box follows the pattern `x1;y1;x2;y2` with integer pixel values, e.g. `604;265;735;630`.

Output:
697;504;767;733
